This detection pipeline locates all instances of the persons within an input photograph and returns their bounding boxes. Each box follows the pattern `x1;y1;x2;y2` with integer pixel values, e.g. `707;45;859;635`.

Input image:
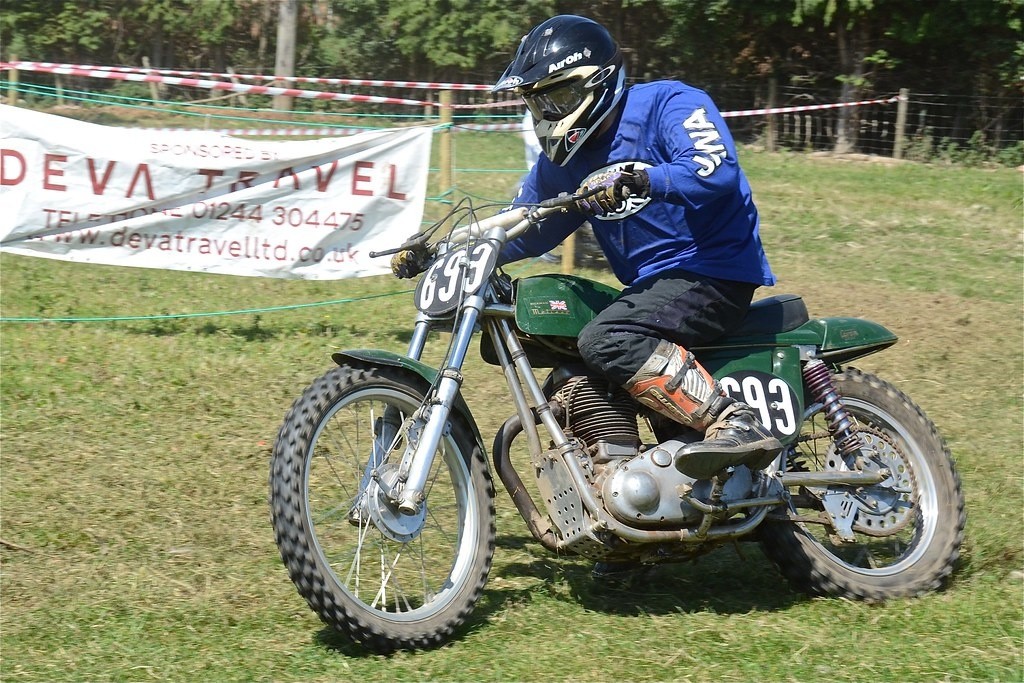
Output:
389;15;782;581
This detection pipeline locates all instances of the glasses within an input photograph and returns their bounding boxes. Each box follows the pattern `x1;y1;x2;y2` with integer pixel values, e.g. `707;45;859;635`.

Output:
521;76;584;121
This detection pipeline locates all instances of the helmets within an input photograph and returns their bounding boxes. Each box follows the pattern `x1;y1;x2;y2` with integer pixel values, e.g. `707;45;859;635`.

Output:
490;15;627;167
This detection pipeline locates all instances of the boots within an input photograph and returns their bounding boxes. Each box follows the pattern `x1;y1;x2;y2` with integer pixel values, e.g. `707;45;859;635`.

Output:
623;340;784;479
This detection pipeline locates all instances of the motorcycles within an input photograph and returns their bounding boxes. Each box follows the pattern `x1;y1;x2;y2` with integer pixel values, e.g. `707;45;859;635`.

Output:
267;161;969;648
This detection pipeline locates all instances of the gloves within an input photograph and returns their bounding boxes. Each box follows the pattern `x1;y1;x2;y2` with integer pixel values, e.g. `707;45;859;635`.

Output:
391;243;436;278
578;163;649;216
